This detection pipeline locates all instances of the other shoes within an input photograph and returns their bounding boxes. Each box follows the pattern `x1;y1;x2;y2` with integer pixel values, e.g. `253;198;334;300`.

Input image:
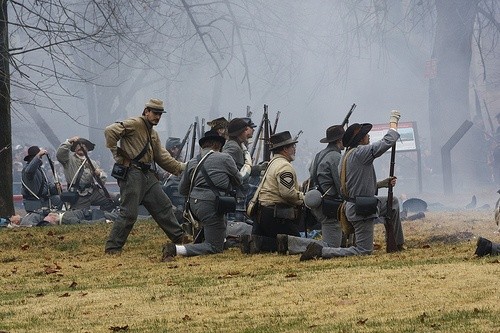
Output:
300;242;323;261
276;234;288;256
162;243;177;260
240;233;263;254
106;247;122;256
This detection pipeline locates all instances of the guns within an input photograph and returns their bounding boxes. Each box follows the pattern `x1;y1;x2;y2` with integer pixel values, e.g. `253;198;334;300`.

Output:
263;103;282;166
250;113;264;157
342;104;356;128
161;124;194;185
386;138;397;252
184;140;189;164
247;104;252;118
78;141;114;205
191;120;198;159
202;117;205;138
45;153;68;212
227;112;234;121
293;129;305;141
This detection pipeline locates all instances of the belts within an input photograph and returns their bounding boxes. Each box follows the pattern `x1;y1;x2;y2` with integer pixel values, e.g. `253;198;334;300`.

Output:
197;184;227;192
345;197;355;203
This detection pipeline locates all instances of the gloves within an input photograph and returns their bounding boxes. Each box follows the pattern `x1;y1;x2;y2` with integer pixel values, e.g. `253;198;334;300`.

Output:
390;110;401;123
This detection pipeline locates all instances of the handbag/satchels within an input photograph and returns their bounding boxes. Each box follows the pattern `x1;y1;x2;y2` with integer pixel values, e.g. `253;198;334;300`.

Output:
216;196;236;213
323;199;342;220
60;192;79;203
257;205;275;230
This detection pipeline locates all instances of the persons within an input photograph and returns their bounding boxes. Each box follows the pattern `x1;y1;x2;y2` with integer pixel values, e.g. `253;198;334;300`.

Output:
22;146;63;214
161;130;243;261
277;125;381;256
300;109;406;262
36;209;118;226
240;131;318;254
163;137;187;222
56;137;117;212
207;117;263;221
12;144;66;195
105;98;193;254
5;208;41;227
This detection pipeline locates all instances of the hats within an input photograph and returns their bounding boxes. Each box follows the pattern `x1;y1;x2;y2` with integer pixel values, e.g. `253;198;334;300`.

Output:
71;138;94;151
342;123;372;148
269;131;298;149
242;118;257;128
225;118;247;136
199;131;226;148
211;117;229;130
320;125;346;143
145;98;167;114
23;146;40;161
